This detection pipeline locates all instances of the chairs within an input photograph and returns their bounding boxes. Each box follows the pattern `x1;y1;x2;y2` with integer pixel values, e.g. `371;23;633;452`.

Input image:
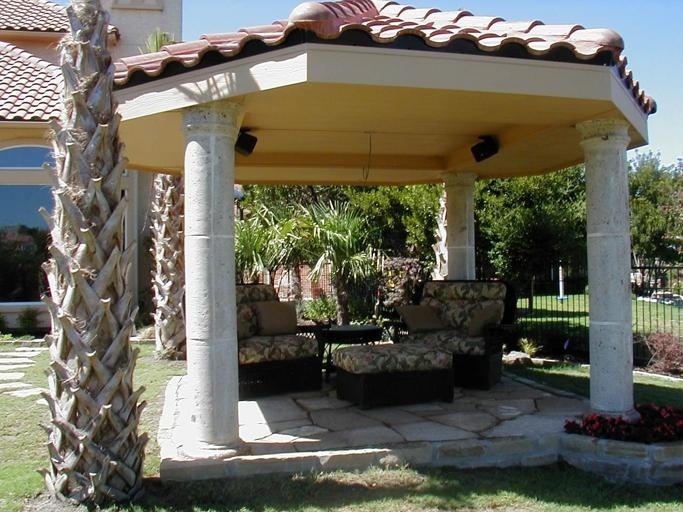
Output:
403;279;517;391
236;281;323;400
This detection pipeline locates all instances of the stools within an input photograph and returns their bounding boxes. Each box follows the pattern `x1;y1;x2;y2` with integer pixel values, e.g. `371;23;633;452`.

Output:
333;342;455;409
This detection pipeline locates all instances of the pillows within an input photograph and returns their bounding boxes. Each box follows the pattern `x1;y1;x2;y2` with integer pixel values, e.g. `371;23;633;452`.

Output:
236;303;257;339
250;300;299;336
468;304;503;337
400;305;444;333
